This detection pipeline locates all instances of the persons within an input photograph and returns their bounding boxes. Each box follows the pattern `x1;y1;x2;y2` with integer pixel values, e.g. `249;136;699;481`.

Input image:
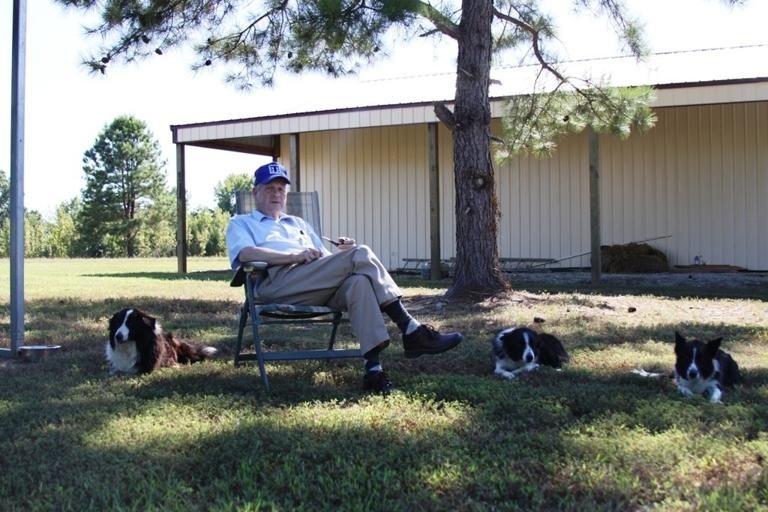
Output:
227;162;461;395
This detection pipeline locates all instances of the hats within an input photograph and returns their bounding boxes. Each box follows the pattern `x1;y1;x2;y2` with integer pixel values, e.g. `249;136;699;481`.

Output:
254;162;291;185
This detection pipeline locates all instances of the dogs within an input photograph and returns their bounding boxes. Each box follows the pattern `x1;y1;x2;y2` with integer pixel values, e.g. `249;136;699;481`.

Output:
493;323;570;382
673;329;744;403
105;306;220;377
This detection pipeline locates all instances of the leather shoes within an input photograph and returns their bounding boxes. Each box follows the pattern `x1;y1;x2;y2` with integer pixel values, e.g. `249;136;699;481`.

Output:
364;370;392;395
402;325;463;360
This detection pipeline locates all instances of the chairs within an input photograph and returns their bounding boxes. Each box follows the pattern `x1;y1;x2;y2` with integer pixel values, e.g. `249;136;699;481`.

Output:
229;191;366;395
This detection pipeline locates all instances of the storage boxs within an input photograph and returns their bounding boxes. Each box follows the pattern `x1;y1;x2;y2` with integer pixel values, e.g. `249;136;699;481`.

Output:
421;261;450;281
